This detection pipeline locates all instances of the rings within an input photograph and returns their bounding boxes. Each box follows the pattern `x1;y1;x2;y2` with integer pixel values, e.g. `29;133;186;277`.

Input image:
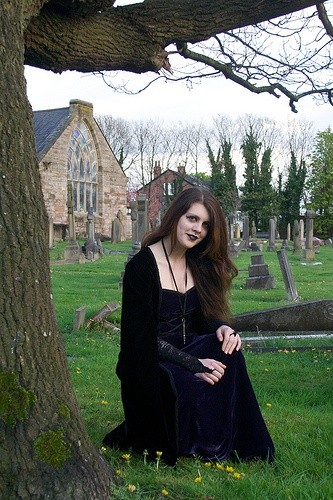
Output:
229;331;237;337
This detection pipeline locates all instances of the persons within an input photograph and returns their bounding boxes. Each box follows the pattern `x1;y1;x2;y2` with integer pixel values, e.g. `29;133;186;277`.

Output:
101;188;277;466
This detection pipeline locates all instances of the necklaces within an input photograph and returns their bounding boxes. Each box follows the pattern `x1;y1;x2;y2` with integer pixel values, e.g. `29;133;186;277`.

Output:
161;238;188;345
